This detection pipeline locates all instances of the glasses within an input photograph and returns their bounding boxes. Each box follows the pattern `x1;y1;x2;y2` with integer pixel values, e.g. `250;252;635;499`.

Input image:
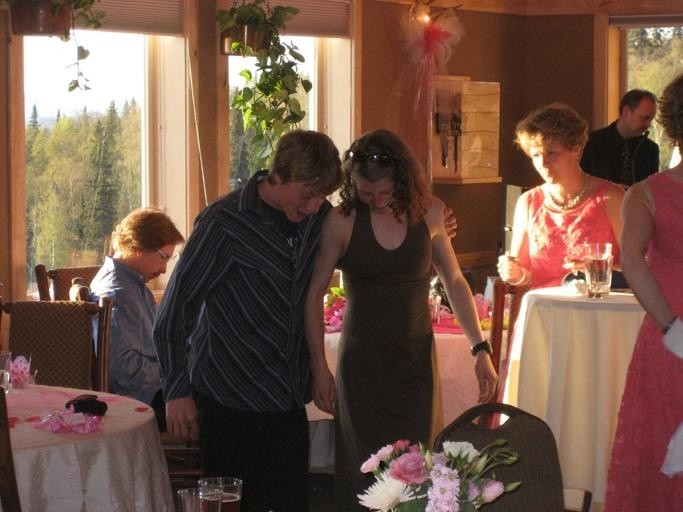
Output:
155;247;175;265
345;149;398;165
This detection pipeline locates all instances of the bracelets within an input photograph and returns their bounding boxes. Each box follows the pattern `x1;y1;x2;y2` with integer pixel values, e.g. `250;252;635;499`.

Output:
508;268;526;286
663;317;678;335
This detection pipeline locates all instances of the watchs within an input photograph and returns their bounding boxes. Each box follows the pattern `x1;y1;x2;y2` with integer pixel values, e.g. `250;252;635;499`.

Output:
470;339;493;356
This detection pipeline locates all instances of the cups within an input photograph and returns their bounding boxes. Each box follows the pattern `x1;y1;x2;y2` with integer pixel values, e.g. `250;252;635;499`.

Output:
428;294;441;326
174;477;243;512
0;351;11;395
582;240;613;299
484;275;500;308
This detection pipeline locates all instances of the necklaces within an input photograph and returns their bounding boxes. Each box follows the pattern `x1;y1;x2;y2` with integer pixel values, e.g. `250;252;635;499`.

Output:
543;174;589;210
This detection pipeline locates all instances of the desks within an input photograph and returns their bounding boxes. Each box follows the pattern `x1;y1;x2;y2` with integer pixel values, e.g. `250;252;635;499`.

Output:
504;290;646;512
6;383;175;512
305;328;493;474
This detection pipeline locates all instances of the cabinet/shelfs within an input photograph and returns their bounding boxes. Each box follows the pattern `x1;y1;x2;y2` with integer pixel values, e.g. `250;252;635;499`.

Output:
435;81;502;185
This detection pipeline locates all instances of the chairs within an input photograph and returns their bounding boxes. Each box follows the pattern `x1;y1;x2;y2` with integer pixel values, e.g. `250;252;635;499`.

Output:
436;402;564;512
493;280;555;384
1;297;115;394
37;263;101;299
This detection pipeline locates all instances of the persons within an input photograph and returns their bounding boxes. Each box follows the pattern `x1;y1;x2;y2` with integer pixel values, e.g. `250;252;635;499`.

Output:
580;89;659;187
302;128;499;512
151;128;457;512
604;75;683;512
496;102;626;292
88;205;187;432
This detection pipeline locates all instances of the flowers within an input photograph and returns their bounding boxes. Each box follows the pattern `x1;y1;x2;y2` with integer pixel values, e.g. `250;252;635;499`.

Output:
321;286;347;333
356;436;524;512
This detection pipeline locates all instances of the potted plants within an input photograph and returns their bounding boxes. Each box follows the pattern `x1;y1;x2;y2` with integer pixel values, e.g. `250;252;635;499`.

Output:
214;0;313;169
4;0;106;92
